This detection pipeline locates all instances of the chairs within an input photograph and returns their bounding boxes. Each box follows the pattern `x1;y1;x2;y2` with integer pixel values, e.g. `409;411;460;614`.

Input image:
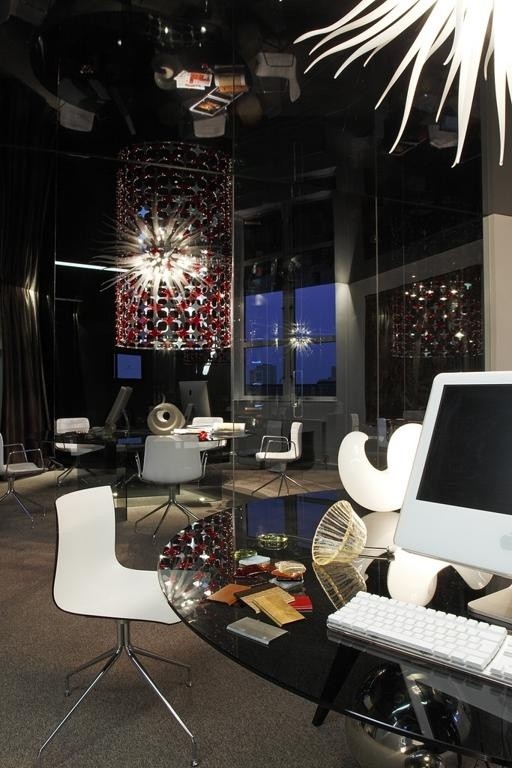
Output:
187;416;227;486
251;421;311;498
334;411;391;459
134;434;202;538
38;485;212;766
54;416;99;486
1;435;47;529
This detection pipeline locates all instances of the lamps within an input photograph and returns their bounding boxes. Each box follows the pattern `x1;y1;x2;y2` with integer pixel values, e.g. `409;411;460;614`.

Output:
84;136;237;354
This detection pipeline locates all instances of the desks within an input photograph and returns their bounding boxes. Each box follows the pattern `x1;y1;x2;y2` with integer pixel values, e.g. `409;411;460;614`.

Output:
53;425;251;499
154;487;512;768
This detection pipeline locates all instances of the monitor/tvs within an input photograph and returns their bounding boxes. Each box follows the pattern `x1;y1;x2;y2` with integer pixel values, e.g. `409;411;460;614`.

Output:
114;353;142;384
178;381;212;425
393;371;512;624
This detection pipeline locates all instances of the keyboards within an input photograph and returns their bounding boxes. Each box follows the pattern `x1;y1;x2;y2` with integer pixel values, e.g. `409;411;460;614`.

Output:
326;591;512;688
